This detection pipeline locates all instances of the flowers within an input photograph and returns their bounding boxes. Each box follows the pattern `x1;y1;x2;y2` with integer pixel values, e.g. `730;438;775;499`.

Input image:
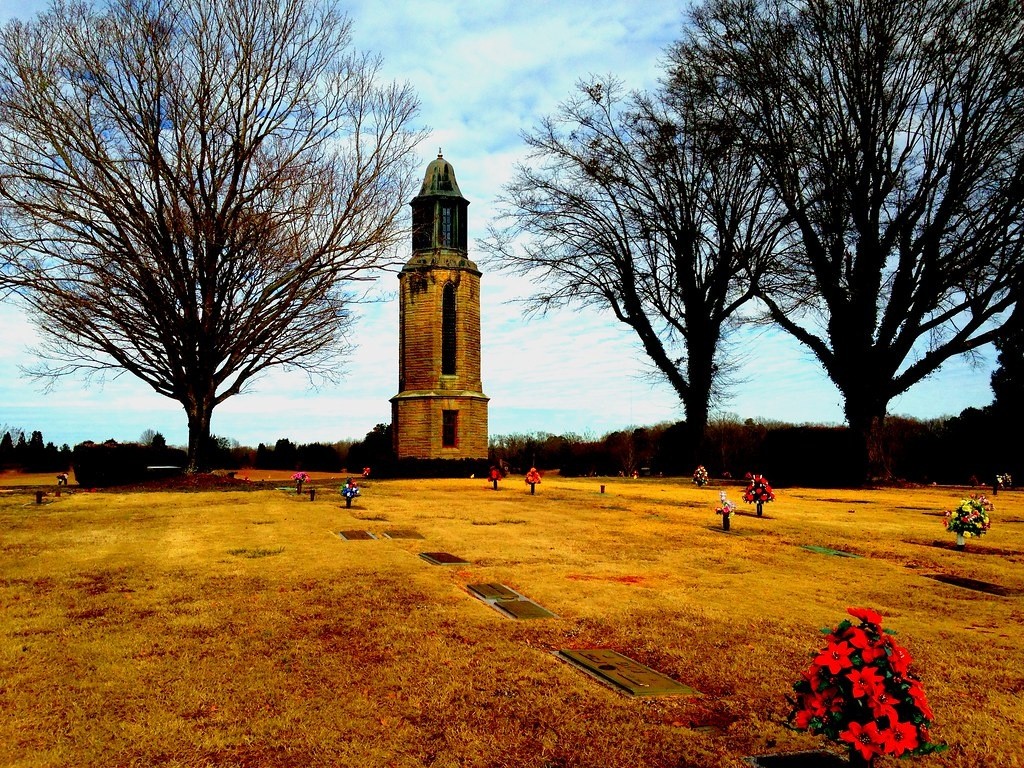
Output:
742;472;775;505
487;466;502;482
722;472;732;479
339;477;361;498
994;473;1013;490
290;472;312;485
632;470;639;477
715;491;737;517
525;467;541;484
943;493;996;538
787;607;948;762
693;464;710;485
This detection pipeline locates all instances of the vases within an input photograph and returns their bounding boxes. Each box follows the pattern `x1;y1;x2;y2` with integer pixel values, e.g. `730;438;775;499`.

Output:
757;504;762;517
849;746;875;768
346;497;351;508
310;490;315;501
494;480;497;490
634;475;637;479
722;514;730;531
956;532;965;546
297;484;301;494
993;487;997;495
698;482;703;487
531;484;535;494
